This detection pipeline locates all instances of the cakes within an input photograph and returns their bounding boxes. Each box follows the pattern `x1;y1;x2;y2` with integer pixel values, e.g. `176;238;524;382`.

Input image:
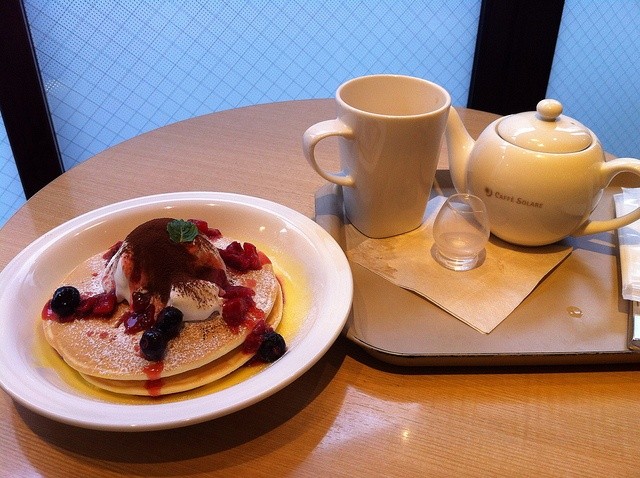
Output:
42;217;288;395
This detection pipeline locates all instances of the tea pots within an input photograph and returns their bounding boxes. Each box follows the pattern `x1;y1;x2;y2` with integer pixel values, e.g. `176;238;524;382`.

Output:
443;99;640;246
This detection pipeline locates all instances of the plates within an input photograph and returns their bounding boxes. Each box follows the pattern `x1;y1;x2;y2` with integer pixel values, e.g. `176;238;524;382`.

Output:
0;190;353;431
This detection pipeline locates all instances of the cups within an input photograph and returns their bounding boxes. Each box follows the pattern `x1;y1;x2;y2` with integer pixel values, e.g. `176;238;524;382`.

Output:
302;74;450;238
432;194;491;271
